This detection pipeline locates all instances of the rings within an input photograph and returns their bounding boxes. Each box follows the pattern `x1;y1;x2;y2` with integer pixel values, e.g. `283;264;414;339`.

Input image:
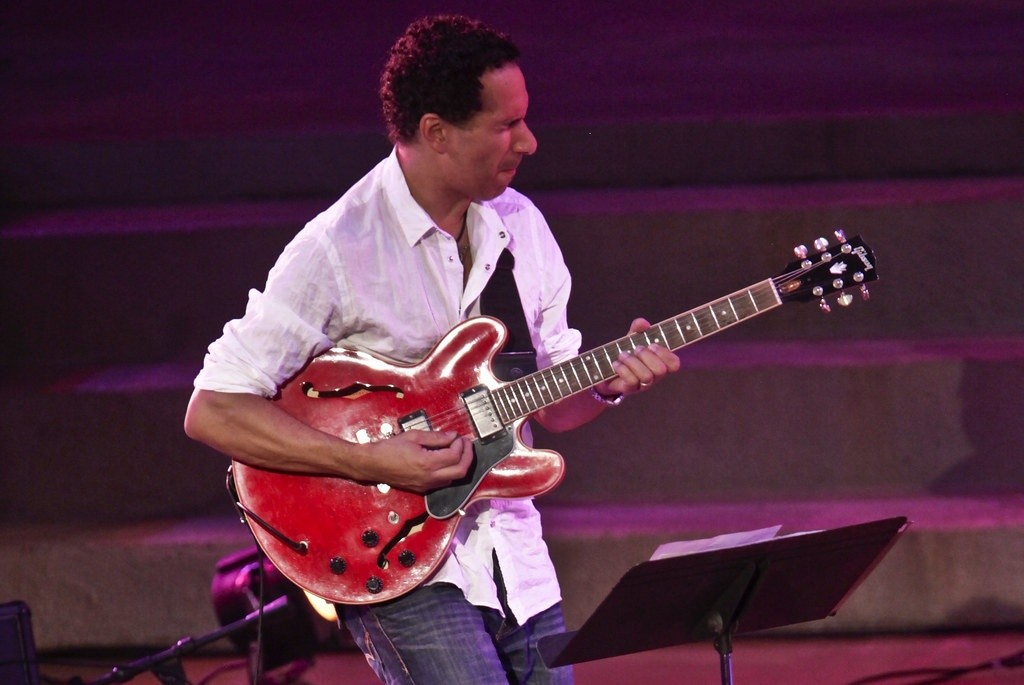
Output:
640;377;653;387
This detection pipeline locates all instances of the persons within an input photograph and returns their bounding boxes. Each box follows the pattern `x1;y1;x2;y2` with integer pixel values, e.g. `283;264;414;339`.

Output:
181;12;681;685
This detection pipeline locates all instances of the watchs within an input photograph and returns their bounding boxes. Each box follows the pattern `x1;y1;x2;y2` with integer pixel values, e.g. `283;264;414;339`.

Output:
589;386;625;408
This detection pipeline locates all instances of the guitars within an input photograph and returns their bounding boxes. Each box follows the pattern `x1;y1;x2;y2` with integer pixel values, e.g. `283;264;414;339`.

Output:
230;229;880;605
